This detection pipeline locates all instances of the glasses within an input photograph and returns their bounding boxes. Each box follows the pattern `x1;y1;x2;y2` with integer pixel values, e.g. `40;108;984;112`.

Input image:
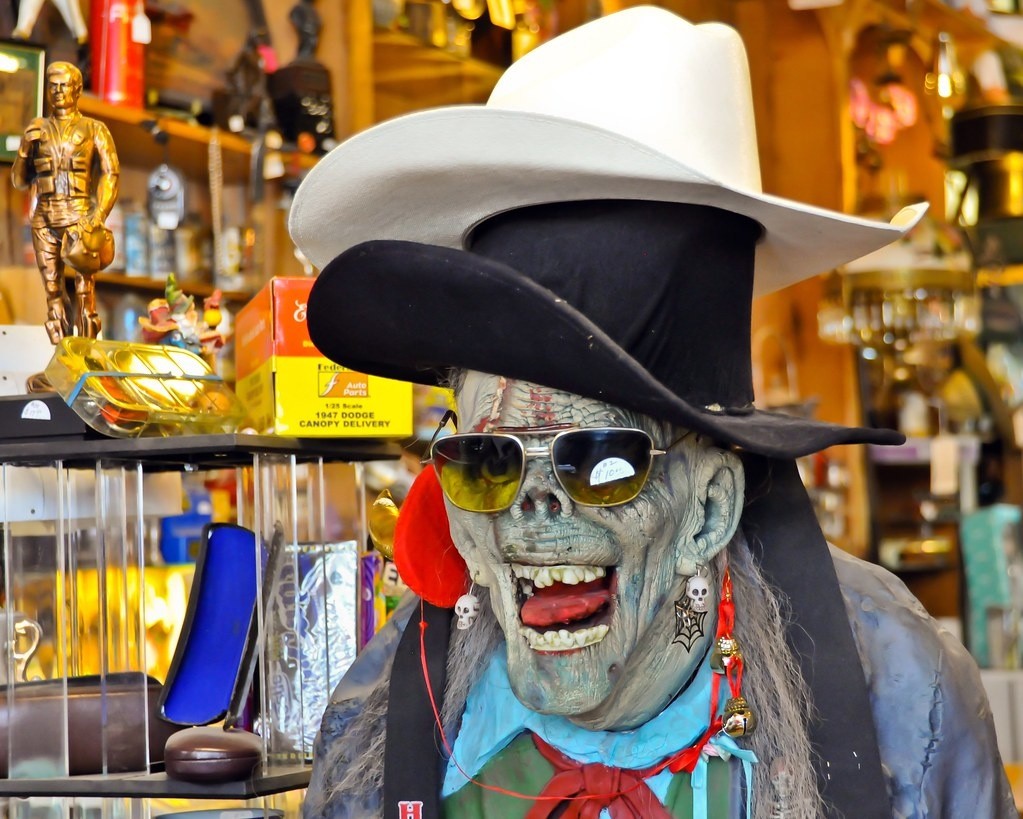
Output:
420;410;694;507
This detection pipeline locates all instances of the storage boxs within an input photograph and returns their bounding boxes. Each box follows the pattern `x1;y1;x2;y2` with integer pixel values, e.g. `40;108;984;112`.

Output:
237;275;415;439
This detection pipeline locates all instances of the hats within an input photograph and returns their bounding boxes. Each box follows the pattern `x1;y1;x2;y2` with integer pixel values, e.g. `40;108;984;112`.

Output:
287;4;930;300
308;199;906;460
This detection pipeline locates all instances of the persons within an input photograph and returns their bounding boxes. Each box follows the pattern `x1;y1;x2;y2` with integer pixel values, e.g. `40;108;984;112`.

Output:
11;61;120;393
233;35;263;95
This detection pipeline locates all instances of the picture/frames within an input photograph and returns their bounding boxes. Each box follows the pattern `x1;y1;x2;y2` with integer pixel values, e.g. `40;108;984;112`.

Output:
0;37;49;167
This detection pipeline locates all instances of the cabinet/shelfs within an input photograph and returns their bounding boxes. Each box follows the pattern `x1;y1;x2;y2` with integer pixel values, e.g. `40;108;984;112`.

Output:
765;0;1023;623
0;0;374;300
